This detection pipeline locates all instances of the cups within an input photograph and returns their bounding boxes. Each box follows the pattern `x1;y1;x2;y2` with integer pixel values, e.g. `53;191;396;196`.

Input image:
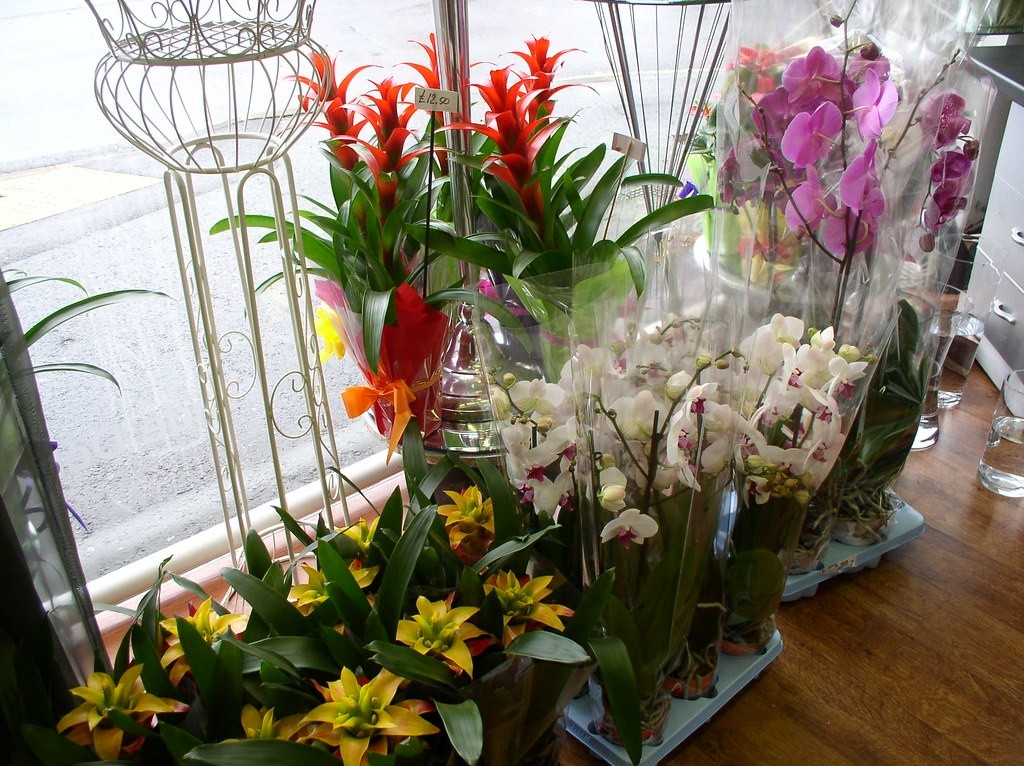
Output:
900;291;935;372
910;283;969;450
977;370;1024;497
935;233;1009;407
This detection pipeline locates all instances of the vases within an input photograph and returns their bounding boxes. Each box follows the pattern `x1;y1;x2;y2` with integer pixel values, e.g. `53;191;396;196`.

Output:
393;443;507;530
693;231;835;346
975;369;1024;498
907;281;972;452
599;697;671;748
781;549;825;575
832;518;883;545
659;653;719;700
923;234;1011;410
429;654;536;766
720;615;775;657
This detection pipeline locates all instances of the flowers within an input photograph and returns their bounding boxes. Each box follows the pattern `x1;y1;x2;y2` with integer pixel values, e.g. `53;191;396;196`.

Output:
0;0;1024;766
